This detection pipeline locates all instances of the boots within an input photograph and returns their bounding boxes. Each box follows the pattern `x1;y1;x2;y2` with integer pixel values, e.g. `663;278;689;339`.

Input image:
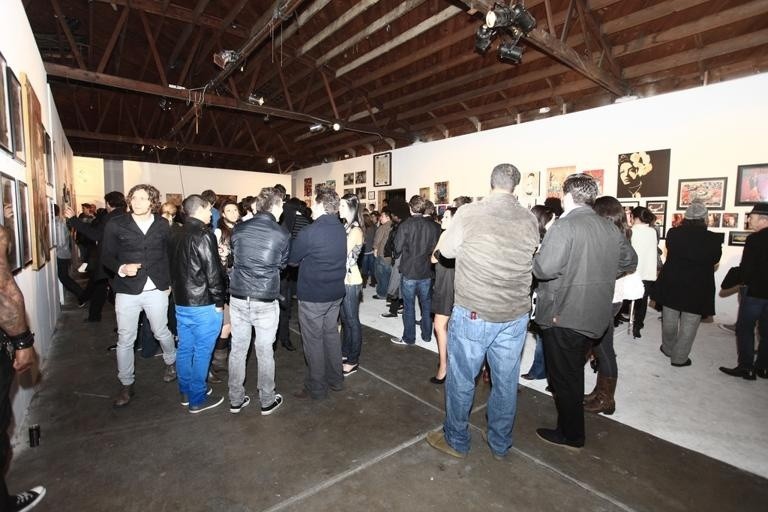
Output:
584;377;617;415
211;348;230;372
583;373;598;400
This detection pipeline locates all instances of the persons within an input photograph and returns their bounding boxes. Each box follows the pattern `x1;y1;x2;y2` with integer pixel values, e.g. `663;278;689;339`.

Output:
718;201;768;381
55;182;365;416
359;165;663;459
723;214;738;227
1;225;48;512
654;202;722;367
616;151;654;199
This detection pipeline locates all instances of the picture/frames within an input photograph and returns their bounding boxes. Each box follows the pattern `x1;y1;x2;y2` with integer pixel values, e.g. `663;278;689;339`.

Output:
728;231;753;246
0;51;57;277
619;201;640;228
676;177;727;210
645;200;667;240
373;152;392;187
735;163;768;206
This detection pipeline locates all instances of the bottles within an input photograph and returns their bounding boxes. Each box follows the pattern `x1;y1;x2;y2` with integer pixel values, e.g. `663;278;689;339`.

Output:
29;423;40;448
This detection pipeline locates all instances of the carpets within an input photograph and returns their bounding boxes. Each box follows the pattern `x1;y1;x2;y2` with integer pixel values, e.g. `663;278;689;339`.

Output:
359;280;768;480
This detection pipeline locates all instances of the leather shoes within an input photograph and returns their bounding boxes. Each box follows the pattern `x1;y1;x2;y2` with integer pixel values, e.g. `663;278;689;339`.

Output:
113;385;137;408
162;362;177;383
280;338;297;352
207;368;224;384
719;362;756;381
520;373;534;380
618;316;630;322
659;343;672;357
293;389;309;401
536;427;585;453
373;294;379;299
671;359;691;366
415;320;421;325
108;344;117;350
755;359;768;379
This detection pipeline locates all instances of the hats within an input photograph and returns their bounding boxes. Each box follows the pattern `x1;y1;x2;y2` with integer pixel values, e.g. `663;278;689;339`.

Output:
745;202;768;216
685;203;708;219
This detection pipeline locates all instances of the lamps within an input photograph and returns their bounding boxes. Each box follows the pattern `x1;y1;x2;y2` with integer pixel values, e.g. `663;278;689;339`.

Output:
475;0;536;65
309;122;324;133
248;89;264;106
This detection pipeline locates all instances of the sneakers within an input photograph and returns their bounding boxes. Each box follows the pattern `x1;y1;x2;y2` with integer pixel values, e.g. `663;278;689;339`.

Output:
426;429;464;458
481;429;506;461
179;387;283;416
380;313;397;317
8;484;47;511
390;336;415;345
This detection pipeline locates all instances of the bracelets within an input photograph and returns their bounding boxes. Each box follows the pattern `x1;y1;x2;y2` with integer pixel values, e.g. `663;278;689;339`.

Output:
7;324;35;351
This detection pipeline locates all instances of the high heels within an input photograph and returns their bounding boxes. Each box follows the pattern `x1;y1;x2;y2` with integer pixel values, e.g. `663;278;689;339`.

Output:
633;332;641;340
429;373;447;384
342;362;359;377
